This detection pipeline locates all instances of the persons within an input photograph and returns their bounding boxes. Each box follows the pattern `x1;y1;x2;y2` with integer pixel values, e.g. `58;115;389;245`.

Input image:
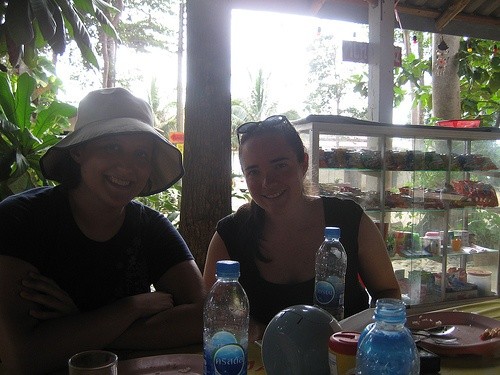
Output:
0;87;203;374
204;115;400;332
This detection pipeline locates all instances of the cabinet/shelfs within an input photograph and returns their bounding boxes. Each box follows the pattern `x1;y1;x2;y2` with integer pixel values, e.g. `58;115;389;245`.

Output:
289;116;500;301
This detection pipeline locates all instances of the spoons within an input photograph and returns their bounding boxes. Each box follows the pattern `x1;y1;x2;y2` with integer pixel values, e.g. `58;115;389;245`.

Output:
407;325;455;335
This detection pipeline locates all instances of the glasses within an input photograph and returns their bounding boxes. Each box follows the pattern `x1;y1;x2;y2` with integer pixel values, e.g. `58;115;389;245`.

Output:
236;114;304;152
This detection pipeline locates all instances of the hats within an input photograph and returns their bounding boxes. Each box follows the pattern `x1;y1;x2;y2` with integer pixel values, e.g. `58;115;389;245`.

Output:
39;88;185;198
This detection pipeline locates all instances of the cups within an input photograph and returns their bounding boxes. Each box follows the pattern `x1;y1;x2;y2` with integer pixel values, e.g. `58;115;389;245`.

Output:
68;351;118;375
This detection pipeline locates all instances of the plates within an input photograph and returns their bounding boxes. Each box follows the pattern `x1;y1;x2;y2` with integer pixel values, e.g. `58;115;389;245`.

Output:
407;311;500;357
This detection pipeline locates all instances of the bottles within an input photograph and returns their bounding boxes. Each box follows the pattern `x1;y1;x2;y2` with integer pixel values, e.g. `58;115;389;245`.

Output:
202;259;250;375
356;297;420;375
316;227;346;320
386;228;492;303
329;331;360;375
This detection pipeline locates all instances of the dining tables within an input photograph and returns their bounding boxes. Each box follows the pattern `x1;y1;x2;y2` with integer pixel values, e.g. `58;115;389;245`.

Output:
244;299;500;375
